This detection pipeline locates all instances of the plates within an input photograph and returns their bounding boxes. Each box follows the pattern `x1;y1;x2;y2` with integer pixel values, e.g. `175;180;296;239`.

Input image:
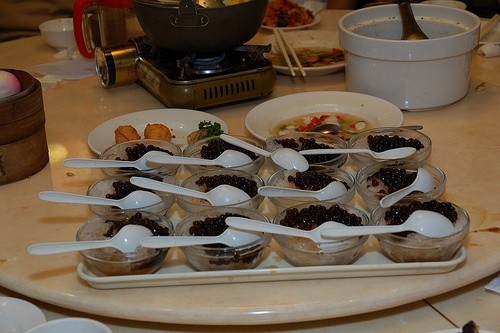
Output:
258;30;345;78
479;20;500;45
244;91;406;141
0;295;46;333
259;9;322;31
86;109;229;154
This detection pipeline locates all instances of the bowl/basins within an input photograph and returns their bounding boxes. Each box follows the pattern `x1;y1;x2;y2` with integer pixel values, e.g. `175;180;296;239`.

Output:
39;18;76;51
419;0;466;10
73;127;470;276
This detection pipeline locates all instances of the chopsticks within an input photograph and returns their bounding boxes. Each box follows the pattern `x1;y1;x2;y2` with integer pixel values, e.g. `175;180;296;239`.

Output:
273;28;306;77
479;14;500;40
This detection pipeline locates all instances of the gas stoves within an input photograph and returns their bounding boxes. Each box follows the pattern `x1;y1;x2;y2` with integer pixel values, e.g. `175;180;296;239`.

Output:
93;36;275;110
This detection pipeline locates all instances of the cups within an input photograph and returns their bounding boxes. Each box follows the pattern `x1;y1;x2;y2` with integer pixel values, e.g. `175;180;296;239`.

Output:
338;4;481;113
27;317;112;333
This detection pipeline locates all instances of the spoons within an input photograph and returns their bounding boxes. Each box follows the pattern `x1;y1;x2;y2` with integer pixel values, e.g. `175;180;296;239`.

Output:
28;133;454;257
397;0;429;41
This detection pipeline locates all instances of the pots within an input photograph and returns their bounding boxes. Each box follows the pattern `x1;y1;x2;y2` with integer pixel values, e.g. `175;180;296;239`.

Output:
131;0;269;55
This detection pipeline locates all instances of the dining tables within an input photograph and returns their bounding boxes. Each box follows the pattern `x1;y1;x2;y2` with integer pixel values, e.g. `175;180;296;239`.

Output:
1;8;500;333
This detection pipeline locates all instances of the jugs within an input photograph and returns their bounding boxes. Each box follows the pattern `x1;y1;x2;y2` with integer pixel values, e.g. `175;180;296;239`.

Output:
72;0;144;59
